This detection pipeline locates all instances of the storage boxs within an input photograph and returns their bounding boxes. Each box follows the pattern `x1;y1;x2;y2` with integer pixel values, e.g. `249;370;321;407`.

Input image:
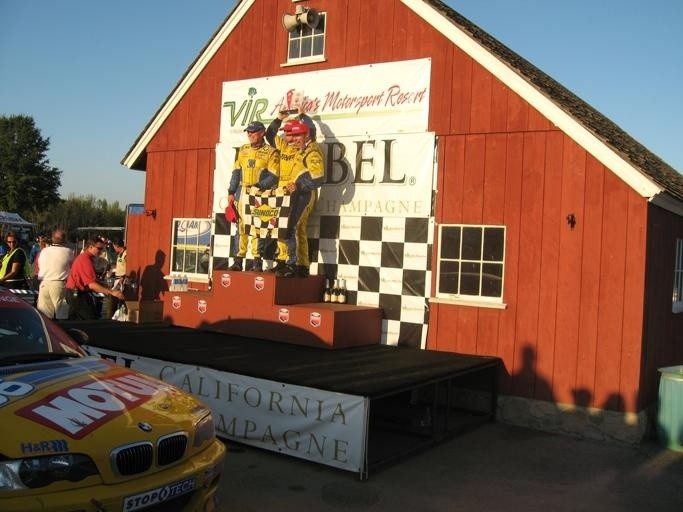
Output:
123;299;164;325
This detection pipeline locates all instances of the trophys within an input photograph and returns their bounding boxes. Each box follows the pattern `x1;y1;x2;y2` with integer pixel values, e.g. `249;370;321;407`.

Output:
278;88;297;115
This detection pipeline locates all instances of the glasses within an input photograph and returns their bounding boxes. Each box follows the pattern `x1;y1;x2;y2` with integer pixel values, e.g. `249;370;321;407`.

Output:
7;241;16;243
96;246;103;250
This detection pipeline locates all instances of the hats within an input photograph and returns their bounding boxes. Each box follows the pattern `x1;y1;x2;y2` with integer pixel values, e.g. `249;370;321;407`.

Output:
225;202;239;223
244;121;264;132
280;120;308;135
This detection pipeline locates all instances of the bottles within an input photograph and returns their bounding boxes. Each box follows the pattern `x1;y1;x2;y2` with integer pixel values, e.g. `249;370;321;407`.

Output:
323;279;346;304
171;274;188;292
119;302;127;323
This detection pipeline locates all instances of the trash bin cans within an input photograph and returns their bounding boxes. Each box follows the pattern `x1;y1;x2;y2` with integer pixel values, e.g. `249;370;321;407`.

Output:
655;365;683;453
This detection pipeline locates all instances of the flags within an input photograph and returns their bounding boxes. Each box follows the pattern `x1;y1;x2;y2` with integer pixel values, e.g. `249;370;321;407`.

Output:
241;186;291;240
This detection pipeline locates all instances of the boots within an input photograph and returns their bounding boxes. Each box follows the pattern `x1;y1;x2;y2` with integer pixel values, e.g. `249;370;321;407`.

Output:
251;257;308;278
226;256;243;271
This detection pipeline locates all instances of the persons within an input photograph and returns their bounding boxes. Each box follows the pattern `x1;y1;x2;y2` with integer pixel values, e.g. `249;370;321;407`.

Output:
274;123;325;278
224;120;279;273
0;228;127;321
266;102;317;273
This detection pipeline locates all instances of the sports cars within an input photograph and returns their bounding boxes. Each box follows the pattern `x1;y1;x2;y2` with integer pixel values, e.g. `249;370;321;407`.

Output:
1;287;226;512
